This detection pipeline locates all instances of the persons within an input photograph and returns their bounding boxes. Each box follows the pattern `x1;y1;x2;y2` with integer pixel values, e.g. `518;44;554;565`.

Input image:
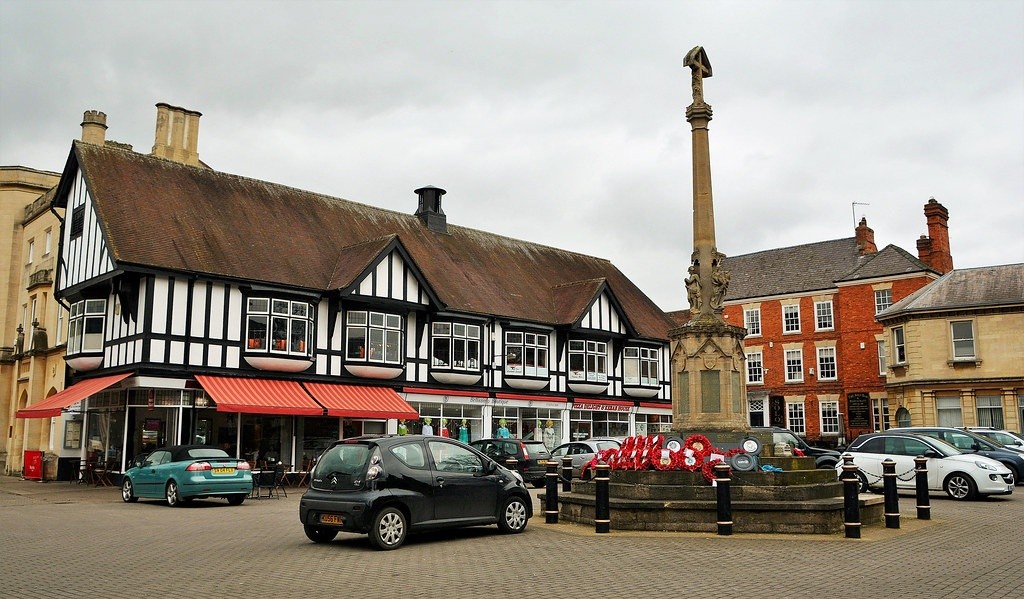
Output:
457;419;468;445
438;418;449;438
398;419;408;435
533;419;543;441
543;420;555;449
421;418;434;436
496;419;510;439
684;266;703;312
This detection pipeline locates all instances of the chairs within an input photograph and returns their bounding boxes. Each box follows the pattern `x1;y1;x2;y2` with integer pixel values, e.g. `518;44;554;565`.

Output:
69;458;116;487
246;460;294;500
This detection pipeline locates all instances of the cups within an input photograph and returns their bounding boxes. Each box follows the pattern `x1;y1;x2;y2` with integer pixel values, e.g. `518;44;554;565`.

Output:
267;456;283;465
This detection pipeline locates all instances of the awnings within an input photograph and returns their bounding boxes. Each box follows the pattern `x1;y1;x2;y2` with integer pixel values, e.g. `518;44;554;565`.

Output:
302;381;419;420
194;374;323;416
15;372;134;418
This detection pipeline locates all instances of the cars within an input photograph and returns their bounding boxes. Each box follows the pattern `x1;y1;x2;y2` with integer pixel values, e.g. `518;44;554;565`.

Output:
548;439;622;485
835;432;1015;501
299;433;534;552
120;445;253;508
749;425;842;470
950;425;1024;463
886;426;1024;487
585;436;648;451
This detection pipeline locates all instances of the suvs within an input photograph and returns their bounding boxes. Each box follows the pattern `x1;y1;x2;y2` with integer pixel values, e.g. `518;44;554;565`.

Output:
467;438;556;489
